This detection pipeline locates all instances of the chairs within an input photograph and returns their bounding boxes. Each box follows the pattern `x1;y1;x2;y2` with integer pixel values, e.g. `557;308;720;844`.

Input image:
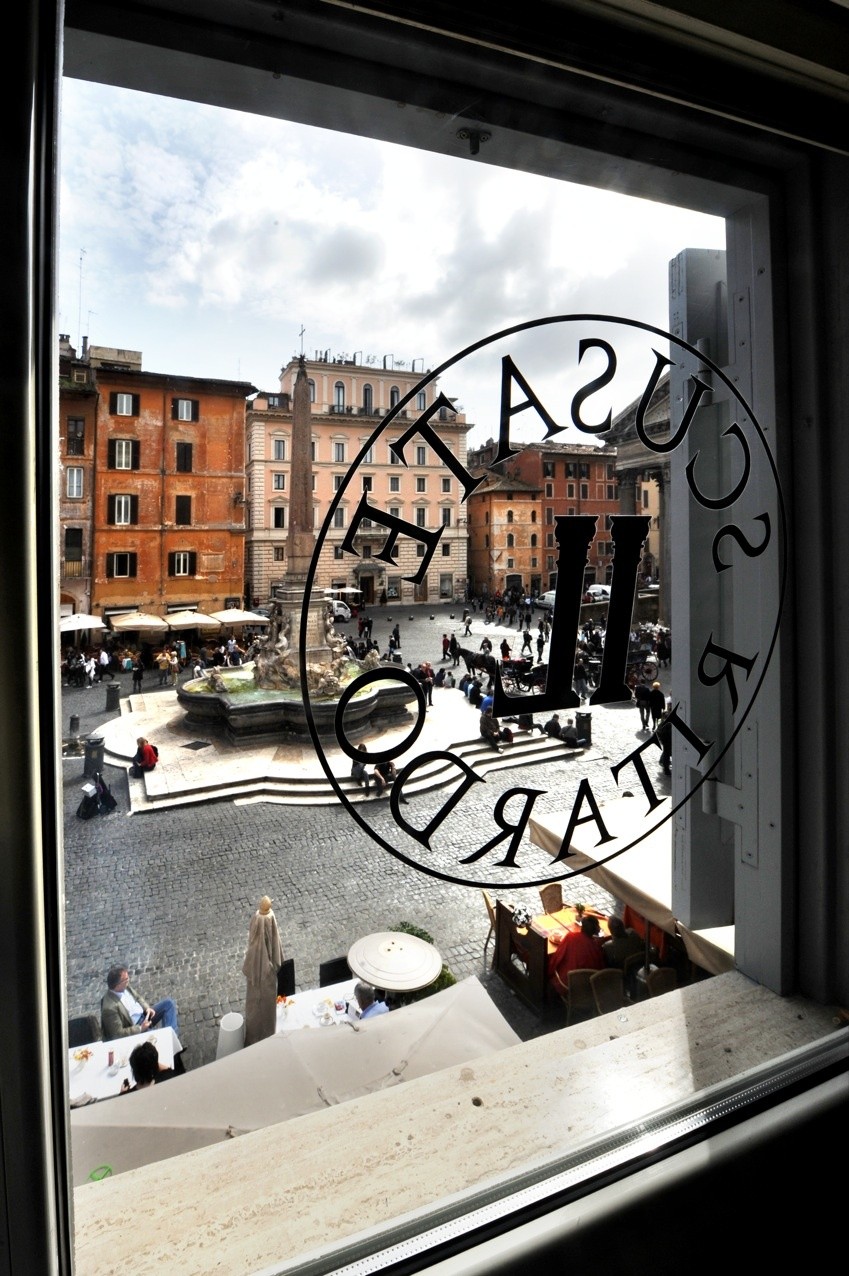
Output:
68;1016;101;1048
481;889;519;961
319;956;353;988
556;950;680;1026
539;884;572;915
278;959;296;998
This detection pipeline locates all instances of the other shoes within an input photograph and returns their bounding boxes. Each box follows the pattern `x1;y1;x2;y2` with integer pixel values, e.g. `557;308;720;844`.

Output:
498;748;504;753
400;800;409;804
366;791;369;797
376;790;383;796
357;781;362;786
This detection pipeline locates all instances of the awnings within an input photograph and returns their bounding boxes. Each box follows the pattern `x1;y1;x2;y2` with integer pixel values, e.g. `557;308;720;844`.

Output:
69;973;523;1202
529;795;736;975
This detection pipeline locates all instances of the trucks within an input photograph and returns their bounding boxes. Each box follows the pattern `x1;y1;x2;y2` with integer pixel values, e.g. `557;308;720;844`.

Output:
582;590;603;602
326;600;351;623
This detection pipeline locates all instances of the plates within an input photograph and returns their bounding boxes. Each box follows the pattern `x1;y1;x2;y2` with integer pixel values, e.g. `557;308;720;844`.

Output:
320;1015;333;1025
549;933;565;945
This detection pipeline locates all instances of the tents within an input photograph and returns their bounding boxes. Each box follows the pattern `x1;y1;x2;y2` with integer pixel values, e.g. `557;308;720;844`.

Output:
60;613;106;634
110;612;169;634
209;608;270;627
161;610;222;631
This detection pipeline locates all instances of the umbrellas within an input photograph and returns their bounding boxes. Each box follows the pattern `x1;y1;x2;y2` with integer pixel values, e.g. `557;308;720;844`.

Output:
244;895;284;1047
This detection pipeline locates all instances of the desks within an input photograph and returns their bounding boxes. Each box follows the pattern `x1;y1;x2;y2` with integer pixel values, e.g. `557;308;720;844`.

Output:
508;903;611;974
276;978;375;1033
69;1025;183;1109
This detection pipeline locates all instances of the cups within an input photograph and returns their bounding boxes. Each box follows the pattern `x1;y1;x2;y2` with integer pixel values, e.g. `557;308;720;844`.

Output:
108;1051;114;1066
554;934;562;942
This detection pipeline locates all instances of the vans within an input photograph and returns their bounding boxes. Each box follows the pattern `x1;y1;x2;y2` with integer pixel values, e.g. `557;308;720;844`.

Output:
589;584;612;600
533;591;556;613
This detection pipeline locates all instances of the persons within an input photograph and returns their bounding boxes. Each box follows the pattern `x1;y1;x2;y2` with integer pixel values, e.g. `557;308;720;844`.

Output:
348;742;370;796
374;755;407;805
339;580;672;752
118;1042;181;1097
99;968;187;1052
62;631;268;775
551;911;642;993
354;980;390;1020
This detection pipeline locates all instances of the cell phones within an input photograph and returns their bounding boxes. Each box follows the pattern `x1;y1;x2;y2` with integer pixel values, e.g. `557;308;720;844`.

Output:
124;1079;129;1090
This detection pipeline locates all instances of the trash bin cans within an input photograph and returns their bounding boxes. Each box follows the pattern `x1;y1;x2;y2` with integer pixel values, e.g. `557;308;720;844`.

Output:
107;682;120;711
575;711;592;746
463;609;469;621
83;735;105;778
392;653;402;663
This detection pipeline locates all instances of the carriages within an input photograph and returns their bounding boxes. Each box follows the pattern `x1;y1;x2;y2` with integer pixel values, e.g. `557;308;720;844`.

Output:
449;647;548;692
